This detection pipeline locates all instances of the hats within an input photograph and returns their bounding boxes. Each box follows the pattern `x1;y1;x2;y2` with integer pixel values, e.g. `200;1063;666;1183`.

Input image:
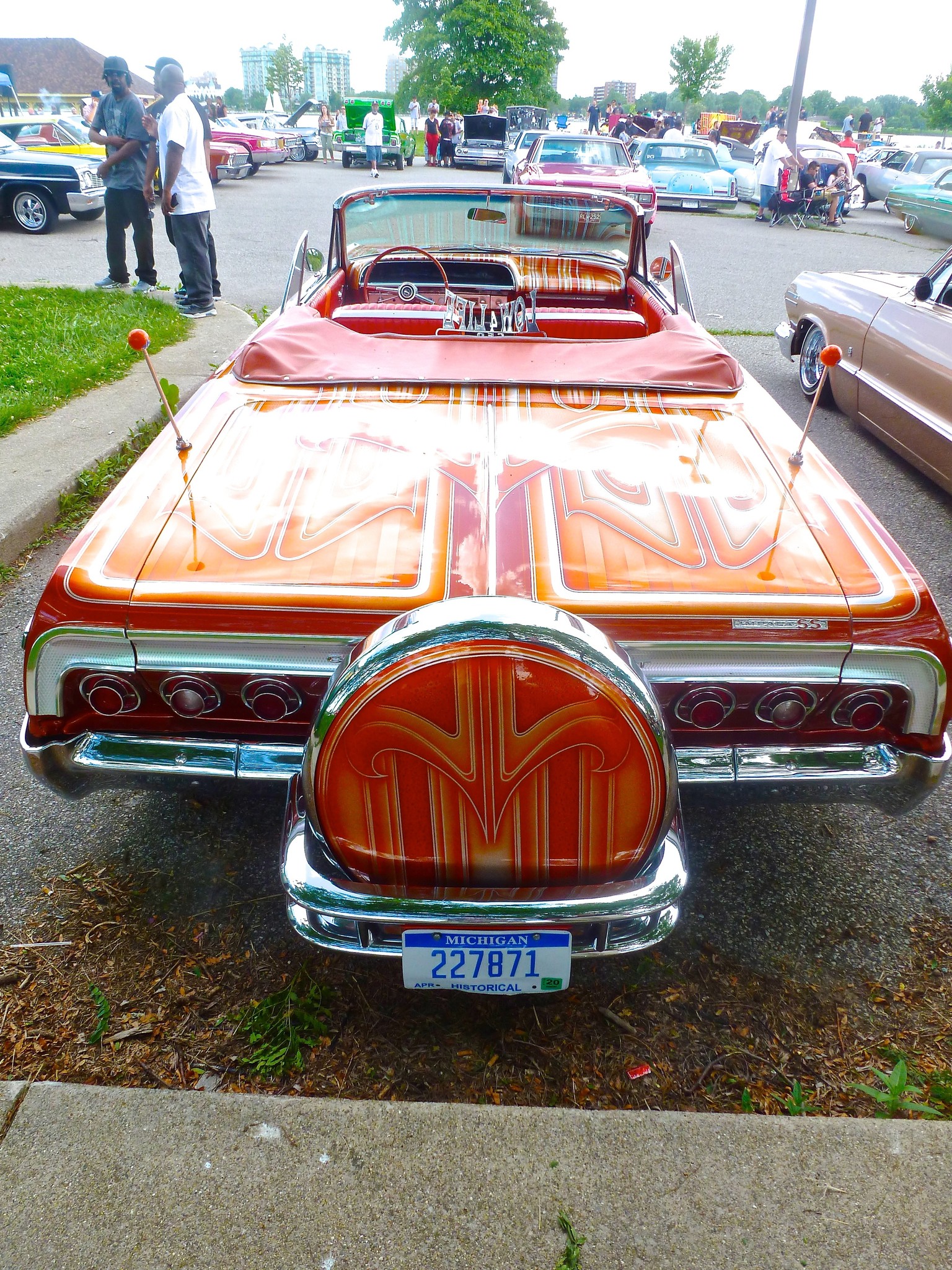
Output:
657;110;662;114
103;56;129;73
654;118;662;123
780;109;783;111
145;57;184;73
672;111;676;114
90;89;104;100
644;107;648;111
372;101;379;106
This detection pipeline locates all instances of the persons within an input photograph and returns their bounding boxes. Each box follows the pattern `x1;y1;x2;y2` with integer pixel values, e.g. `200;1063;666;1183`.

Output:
336;110;341;121
215;96;228;118
600;100;701;151
142;57;223;301
438;111;456;169
318;104;336;164
27;88;160;137
509;108;549;131
799;161;846;228
88;55;157;294
455;114;465;140
204;97;218;123
409;95;422;136
446;114;462;167
755;128;804;223
827;163;851;224
763;106;808;130
362;100;384;178
475;99;484;114
491;104;499;117
427;98;440;118
585;100;602;135
708;121;721;135
336;105;349;130
480;98;490;115
837;109;951;174
142;64;219;319
750;115;761;137
424;110;440;167
708;130;732;160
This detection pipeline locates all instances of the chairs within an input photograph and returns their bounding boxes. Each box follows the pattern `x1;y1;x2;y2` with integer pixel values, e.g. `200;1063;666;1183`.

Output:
794;164;845;226
818;164;848;225
769;176;813;231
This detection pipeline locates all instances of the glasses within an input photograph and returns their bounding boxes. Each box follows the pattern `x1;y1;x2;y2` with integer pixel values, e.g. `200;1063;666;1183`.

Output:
341;108;345;110
779;134;788;137
104;71;127;77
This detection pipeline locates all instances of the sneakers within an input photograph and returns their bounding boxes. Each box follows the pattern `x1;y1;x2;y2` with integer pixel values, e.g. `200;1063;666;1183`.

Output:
831;190;846;197
370;170;379;178
331;157;335;163
323;159;327;164
176;299;217;319
771;218;784;224
174;287;188;299
211;295;221;300
94;276;130;289
826;220;841;228
133;280;157;294
755;214;771;222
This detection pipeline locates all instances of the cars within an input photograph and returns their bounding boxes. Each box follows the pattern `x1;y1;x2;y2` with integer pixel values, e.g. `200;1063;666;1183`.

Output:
0;86;951;1002
